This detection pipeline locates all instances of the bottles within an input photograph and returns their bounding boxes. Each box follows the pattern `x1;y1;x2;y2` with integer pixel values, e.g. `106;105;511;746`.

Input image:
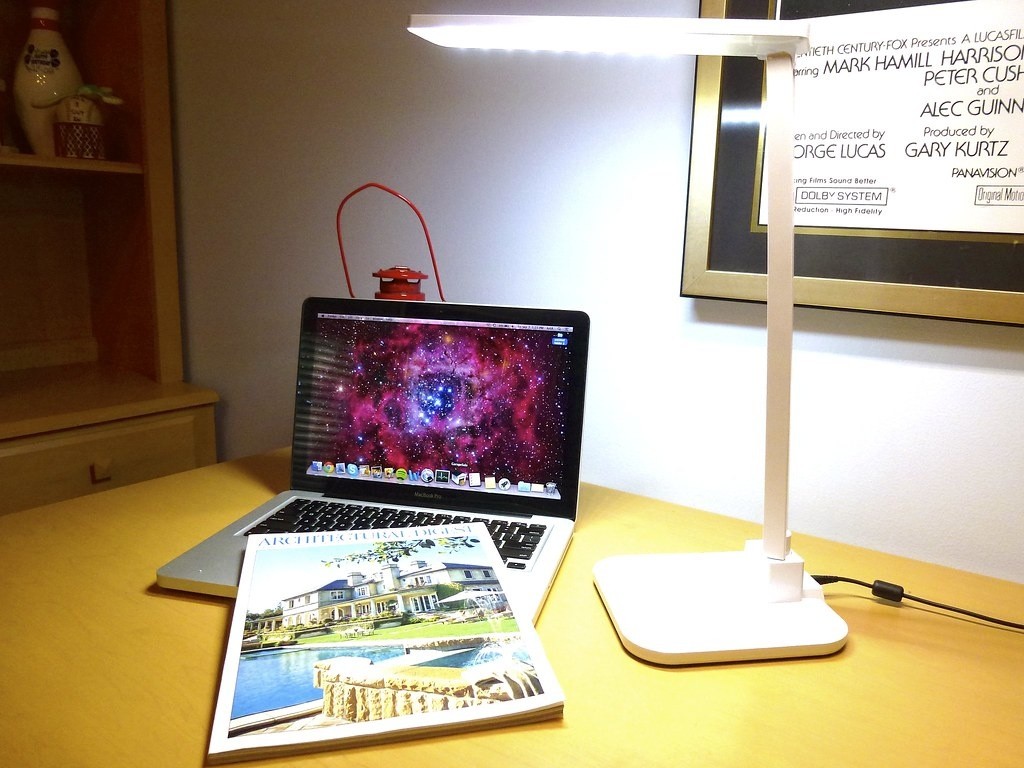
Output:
12;6;83;156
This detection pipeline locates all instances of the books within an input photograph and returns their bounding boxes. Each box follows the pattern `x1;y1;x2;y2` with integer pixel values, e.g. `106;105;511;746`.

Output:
206;523;564;765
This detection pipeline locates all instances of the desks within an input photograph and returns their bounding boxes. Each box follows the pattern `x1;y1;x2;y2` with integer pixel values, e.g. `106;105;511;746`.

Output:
0;446;1024;768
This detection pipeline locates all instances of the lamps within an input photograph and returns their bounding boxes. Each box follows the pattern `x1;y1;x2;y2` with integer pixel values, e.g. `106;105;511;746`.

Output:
408;11;850;667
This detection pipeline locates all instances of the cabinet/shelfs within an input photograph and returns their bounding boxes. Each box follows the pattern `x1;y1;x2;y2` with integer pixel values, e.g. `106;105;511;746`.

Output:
0;0;219;519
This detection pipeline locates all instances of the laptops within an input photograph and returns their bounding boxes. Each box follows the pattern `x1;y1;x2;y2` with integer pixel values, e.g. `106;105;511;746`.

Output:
155;295;590;630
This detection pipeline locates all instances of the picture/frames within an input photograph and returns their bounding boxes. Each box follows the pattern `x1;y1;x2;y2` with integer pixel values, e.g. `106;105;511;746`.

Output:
678;0;1024;329
